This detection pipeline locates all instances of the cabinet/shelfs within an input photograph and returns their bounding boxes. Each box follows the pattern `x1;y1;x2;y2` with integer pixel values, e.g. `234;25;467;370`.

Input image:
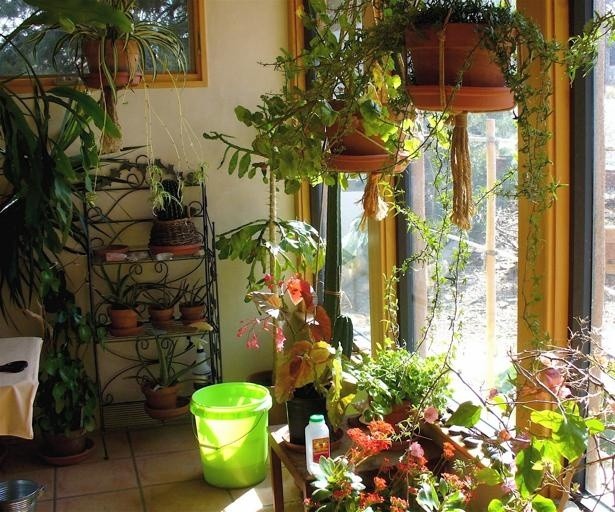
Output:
86;164;224;462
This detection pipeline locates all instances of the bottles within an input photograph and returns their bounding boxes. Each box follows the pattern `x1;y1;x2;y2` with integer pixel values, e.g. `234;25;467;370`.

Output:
305;414;331;476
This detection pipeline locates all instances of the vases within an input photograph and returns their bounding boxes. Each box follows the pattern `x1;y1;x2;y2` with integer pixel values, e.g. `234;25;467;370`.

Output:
285;384;343;444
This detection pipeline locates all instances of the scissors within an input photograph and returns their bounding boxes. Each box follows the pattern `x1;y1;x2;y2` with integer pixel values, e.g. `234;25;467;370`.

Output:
0;361;28;373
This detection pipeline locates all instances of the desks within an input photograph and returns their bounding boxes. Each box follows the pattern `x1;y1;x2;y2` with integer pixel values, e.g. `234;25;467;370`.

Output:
0;337;39;464
266;422;444;508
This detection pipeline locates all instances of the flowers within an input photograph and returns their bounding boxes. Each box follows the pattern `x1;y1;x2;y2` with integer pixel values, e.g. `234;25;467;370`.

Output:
338;407;468;511
233;273;334;407
487;345;614;512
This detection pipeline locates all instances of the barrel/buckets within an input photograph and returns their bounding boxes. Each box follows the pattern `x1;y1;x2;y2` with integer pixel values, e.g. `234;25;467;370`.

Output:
189;381;273;490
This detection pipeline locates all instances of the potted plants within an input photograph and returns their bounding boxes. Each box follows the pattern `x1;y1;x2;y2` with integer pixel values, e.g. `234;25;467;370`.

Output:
343;346;452;449
148;179;203;256
29;273;105;468
137;280;190;321
378;2;615;112
90;273;144;329
121;336;210;415
19;0;206;209
205;2;411;195
176;277;212;320
